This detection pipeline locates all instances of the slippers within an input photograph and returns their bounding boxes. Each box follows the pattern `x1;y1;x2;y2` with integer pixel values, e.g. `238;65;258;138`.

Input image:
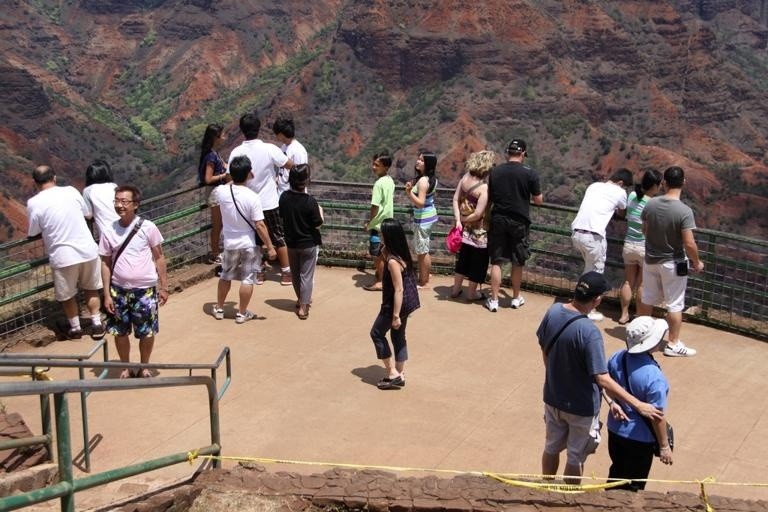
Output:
299;304;310;320
618;313;633;325
467;292;489;301
450;288;464;298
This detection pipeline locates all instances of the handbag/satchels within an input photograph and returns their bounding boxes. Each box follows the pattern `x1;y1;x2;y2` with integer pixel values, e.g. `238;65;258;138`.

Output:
445;225;462;254
622;351;674;458
230;184;265;247
100;216;145;315
677;259;688;276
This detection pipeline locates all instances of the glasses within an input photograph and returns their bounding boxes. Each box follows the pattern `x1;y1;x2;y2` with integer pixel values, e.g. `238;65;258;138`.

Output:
112;198;133;206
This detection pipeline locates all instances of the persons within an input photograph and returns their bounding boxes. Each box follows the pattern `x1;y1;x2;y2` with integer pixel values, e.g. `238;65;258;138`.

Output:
569;167;634;321
484;138;543;313
618;169;664;325
81;158;121;240
363;151;395;292
404;151;439;290
450;150;497;303
98;184;169;379
602;315;675;492
536;271;664;485
226;113;294;285
641;165;704;358
25;163;107;340
197;123;229;264
278;162;325;320
209;154;278;324
272;118;308;199
370;217;421;390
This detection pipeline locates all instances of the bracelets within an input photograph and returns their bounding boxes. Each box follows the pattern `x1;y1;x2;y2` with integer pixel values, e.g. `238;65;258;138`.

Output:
660;445;670;450
608;400;615;406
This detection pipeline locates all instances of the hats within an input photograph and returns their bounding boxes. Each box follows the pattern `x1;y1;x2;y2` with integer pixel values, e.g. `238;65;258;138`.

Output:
625;315;669;354
574;270;612;299
508;138;529;158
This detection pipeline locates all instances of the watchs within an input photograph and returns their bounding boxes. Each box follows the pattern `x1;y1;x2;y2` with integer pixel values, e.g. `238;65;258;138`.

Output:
158;287;169;292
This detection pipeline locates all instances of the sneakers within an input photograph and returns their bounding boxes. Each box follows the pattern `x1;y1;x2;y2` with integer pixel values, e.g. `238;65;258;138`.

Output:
377;375;406;389
280;272;293;285
485;297;500;313
91;325;107;340
210;303;224;319
207;252;223;267
136;368;153;379
663;340;697;356
255;271;266;284
236;309;253;323
510;295;525;308
362;283;383;291
59;326;84;342
586;312;604;320
119;368;133;382
